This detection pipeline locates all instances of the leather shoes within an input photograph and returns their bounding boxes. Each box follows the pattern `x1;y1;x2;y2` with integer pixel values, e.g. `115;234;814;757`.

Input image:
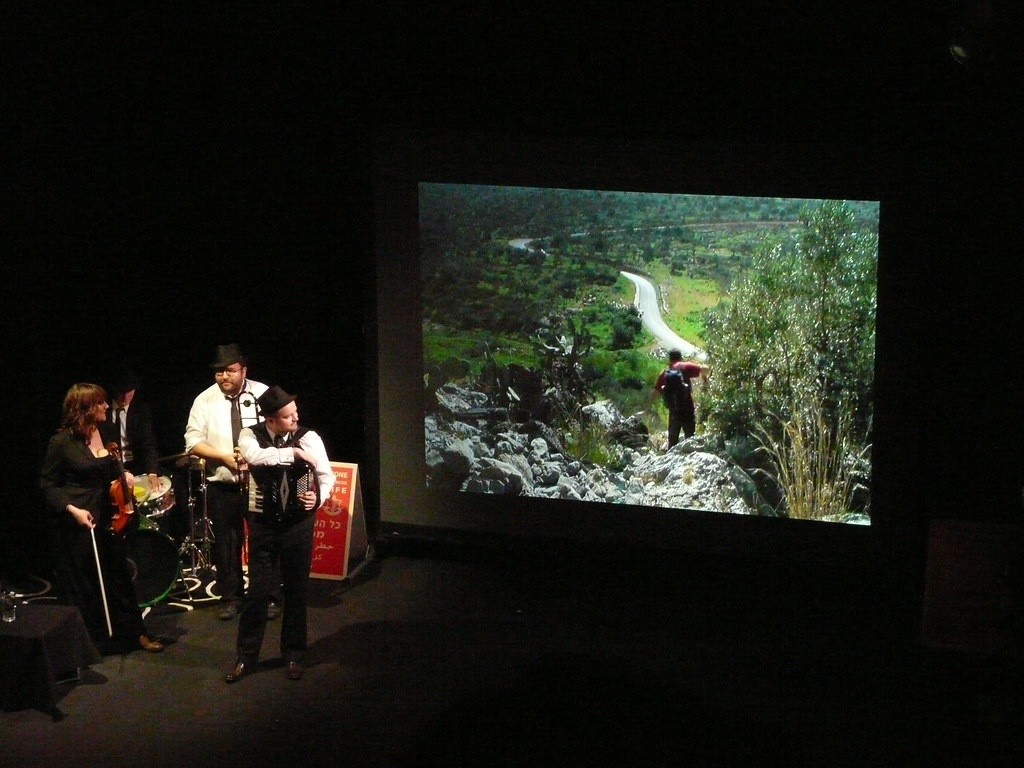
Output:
225;657;247;679
268;601;282;619
287;661;305;678
219;600;241;619
137;632;165;651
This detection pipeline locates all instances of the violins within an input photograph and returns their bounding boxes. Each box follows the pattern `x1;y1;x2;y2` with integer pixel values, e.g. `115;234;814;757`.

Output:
106;441;141;543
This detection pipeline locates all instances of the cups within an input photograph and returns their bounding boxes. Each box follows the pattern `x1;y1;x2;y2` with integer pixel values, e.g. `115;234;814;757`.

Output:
3;594;16;622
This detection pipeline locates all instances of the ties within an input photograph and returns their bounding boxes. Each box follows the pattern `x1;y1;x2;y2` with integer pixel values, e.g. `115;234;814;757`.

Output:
115;408;124;460
272;434;284;513
227;378;246;452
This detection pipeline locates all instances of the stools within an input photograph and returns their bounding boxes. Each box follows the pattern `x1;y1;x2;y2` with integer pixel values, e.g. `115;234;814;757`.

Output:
8;604;101;706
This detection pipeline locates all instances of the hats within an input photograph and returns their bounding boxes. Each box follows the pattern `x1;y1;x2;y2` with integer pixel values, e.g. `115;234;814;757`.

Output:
257;384;297;415
208;343;246;367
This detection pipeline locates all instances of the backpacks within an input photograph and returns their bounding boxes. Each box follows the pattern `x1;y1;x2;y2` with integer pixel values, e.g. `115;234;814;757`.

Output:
661;368;689;411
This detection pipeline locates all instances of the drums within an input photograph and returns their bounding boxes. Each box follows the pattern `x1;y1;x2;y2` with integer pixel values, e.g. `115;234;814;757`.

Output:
132;473;176;519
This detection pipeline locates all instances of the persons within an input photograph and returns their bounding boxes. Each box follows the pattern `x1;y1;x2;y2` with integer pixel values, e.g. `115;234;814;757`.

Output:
183;345;281;619
650;351;709;448
223;384;335;684
101;367;158;478
35;383;164;651
